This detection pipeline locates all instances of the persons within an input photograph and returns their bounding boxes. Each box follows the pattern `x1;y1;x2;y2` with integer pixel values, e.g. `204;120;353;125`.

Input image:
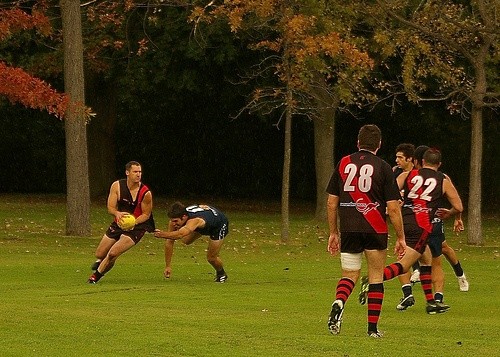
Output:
359;144;468;316
90;161;156;283
325;125;407;339
154;201;229;283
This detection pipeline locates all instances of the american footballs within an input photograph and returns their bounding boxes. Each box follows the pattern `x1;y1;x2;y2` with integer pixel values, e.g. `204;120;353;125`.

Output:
119;214;136;231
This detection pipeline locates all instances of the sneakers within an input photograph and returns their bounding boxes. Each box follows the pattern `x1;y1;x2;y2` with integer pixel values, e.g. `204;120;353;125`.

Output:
367;329;382;337
91;259;101;270
396;295;415;311
457;272;468;292
435;292;443;305
427;304;451;315
327;299;344;335
358;276;369;305
214;269;227;283
410;269;421;283
89;271;104;283
